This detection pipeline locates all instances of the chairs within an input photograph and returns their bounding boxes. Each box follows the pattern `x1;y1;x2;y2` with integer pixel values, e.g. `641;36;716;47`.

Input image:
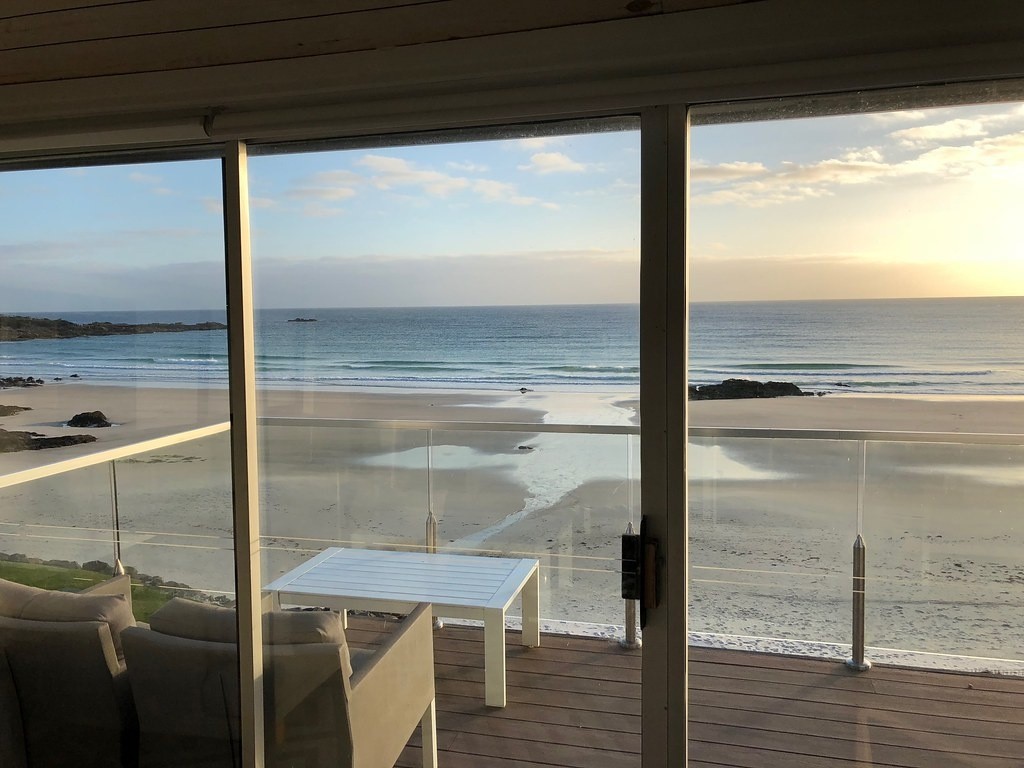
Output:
0;575;438;768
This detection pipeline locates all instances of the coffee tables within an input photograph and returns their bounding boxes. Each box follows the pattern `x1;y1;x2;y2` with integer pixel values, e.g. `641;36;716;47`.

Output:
262;546;540;708
